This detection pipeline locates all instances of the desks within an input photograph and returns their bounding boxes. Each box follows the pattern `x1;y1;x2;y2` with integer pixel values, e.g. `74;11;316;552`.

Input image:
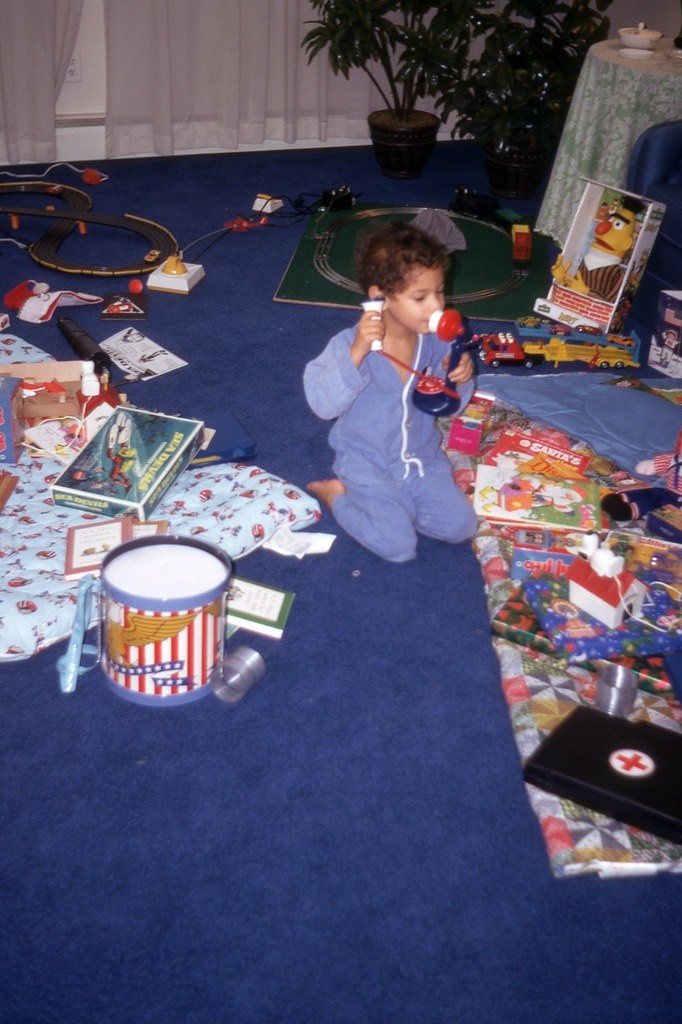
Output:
535;38;682;250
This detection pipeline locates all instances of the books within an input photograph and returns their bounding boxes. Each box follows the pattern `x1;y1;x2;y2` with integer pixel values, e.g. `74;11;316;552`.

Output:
133;518;169;539
469;426;682;850
225;576;296;641
64;516;135;580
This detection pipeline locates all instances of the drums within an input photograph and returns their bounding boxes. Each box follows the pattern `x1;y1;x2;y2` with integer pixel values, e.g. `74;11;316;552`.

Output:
98;532;236;706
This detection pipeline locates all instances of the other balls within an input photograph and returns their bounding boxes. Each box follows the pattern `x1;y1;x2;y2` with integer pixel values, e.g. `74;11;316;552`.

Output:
128;278;145;295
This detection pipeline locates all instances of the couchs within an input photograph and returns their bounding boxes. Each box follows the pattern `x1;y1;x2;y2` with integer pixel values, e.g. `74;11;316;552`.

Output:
626;120;682;328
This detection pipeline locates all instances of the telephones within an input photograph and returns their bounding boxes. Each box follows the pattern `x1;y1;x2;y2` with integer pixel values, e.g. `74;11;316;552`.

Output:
358;297;465;417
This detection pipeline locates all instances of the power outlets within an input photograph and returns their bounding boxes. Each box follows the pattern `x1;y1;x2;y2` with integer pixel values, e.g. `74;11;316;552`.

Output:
61;51;82;83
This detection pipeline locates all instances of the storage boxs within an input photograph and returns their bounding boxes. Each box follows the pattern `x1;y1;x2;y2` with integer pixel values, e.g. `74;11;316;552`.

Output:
648;289;682;379
522;570;682;665
0;376;26;464
448;392;495;453
0;359;87;417
51;405;206;521
489;583;678;704
534;176;667;332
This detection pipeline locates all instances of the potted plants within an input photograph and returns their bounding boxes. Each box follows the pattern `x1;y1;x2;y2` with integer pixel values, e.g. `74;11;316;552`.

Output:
390;0;614;200
301;0;503;181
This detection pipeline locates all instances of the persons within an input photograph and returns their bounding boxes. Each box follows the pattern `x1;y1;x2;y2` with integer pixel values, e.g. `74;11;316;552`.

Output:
302;227;483;564
